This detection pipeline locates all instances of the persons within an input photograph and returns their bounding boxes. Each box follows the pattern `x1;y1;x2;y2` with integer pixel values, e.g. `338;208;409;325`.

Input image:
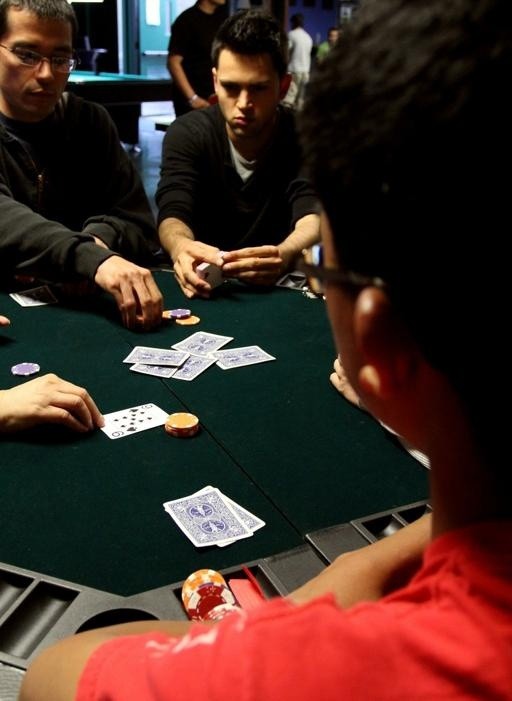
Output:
314;27;338;68
327;354;432;473
154;9;323;299
18;2;508;701
1;373;107;435
281;13;313;112
166;0;227;117
1;0;166;336
1;315;12;326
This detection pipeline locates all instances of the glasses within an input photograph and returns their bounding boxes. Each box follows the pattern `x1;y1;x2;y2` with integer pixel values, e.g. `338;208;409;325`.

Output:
0;43;81;74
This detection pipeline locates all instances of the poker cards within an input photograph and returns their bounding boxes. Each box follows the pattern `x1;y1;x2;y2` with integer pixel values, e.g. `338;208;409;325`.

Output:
99;401;170;441
196;250;229;289
164;485;267;551
122;331;278;383
9;284;59;308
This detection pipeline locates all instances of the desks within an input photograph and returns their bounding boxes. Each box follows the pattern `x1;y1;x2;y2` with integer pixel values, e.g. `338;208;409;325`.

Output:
0;265;434;695
66;68;171;162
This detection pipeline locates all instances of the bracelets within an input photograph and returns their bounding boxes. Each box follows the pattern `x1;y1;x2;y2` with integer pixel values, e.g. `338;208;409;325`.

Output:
188;94;198;105
